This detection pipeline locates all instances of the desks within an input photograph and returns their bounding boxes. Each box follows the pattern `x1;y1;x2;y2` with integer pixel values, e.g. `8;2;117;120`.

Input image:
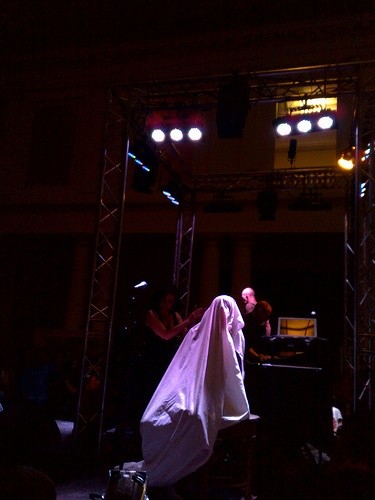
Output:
171;412;262;500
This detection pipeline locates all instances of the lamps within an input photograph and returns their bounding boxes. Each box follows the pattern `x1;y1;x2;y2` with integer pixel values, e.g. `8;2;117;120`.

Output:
146;110;205;142
161;183;183;205
216;75;251;138
337;145;358;171
272;99;336;138
206;195;242;213
288;193;332;213
127;142;157;173
256;189;279;222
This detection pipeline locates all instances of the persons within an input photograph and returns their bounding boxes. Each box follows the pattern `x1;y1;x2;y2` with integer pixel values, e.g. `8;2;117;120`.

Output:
240;287;273;364
142;286;203;375
